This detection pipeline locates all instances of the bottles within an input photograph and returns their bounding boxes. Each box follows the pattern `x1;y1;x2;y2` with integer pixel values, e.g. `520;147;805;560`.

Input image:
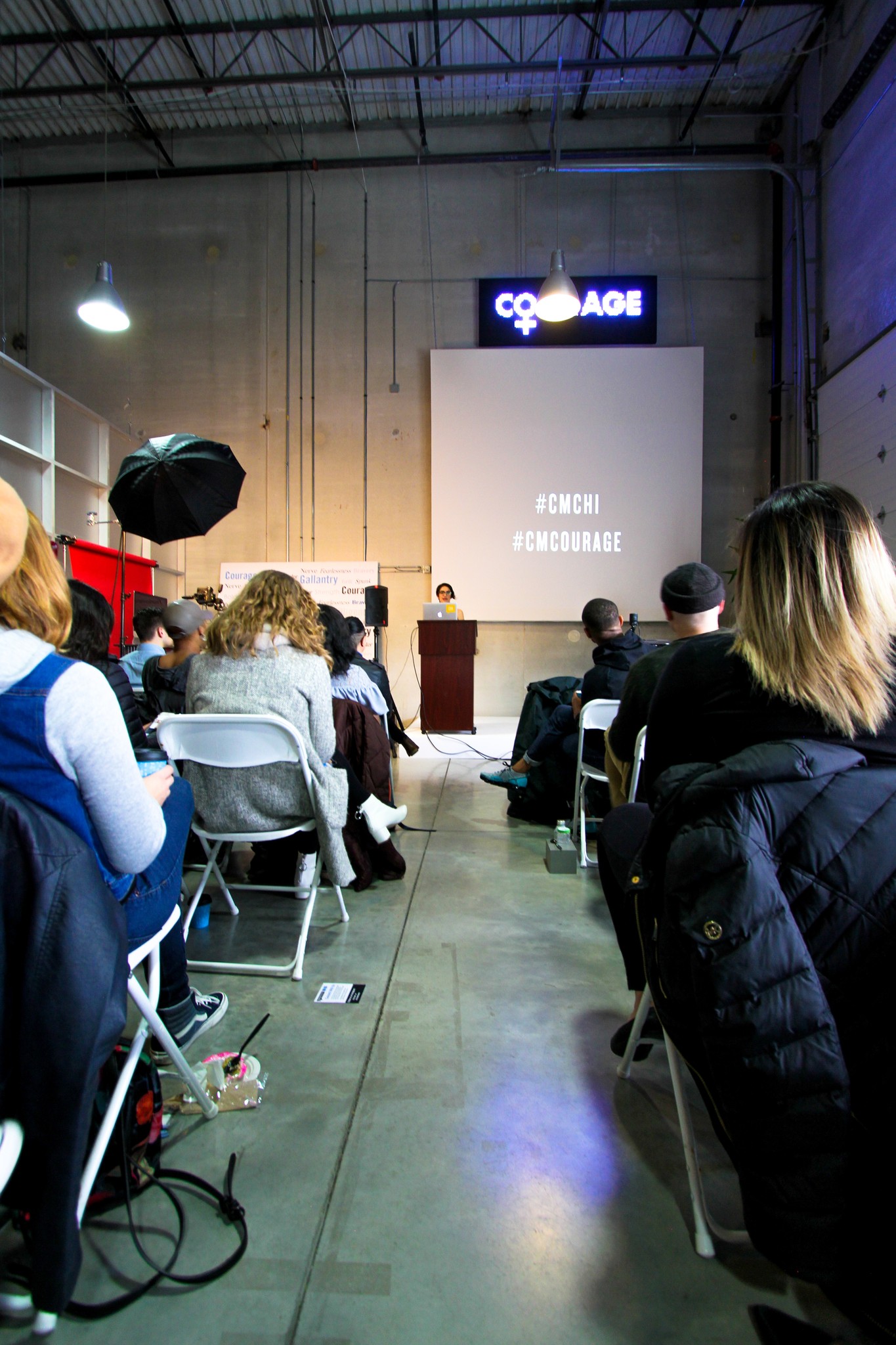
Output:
553;820;566;840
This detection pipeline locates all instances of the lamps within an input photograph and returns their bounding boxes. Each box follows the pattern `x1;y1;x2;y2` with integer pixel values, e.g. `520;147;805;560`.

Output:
534;0;583;322
77;0;131;333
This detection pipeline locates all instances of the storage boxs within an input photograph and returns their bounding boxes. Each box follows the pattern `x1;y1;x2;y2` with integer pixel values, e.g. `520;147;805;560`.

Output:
545;838;577;875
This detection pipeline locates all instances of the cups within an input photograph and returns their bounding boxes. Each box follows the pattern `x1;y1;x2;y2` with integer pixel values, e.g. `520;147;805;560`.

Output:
557;827;571;844
187;894;213;929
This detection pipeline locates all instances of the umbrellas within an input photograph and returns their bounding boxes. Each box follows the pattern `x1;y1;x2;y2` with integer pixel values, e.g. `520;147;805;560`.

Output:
107;434;246;545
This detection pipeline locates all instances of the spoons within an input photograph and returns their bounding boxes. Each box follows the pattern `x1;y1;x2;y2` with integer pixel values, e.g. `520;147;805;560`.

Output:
223;1013;271;1075
550;839;562;850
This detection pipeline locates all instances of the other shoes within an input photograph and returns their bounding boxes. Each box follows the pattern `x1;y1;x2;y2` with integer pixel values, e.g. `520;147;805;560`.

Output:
610;1007;664;1063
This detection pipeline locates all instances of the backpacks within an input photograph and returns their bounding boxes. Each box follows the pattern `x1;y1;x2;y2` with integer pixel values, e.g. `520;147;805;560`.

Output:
87;1039;164;1211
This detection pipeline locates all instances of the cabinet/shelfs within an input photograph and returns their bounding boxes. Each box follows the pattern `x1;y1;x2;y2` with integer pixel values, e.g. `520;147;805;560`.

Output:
0;352;186;602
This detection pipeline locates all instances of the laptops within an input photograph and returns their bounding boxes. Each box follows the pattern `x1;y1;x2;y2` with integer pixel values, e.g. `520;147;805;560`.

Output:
423;603;457;621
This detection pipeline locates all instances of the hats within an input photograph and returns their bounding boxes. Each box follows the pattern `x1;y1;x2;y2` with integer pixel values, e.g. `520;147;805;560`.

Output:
165;598;212;641
0;478;28;586
662;564;724;614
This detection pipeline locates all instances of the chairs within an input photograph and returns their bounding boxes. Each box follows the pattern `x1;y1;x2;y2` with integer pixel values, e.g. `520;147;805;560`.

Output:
0;790;222;1334
156;713;349;980
618;981;748;1256
570;699;620;869
628;725;648;804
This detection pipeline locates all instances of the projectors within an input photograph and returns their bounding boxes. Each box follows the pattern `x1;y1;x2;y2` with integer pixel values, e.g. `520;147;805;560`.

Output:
643;640;671;648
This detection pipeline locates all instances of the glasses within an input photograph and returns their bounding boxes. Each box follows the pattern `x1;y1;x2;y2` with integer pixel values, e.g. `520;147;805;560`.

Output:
440;591;452;596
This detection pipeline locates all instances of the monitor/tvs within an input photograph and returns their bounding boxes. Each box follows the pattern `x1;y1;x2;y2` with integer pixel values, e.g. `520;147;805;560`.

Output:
132;590;168;617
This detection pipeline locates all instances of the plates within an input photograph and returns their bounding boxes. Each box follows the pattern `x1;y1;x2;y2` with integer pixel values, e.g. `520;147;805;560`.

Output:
205;1052;261;1099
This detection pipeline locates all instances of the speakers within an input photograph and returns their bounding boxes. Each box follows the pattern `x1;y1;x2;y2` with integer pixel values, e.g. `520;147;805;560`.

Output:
365;585;388;627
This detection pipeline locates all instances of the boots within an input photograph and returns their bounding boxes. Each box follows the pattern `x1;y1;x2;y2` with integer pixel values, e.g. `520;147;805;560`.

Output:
294;853;321;900
355;794;408;843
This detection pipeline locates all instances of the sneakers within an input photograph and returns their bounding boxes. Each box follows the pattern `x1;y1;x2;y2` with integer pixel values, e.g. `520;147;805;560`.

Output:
480;761;527;791
149;985;229;1065
403;739;419;756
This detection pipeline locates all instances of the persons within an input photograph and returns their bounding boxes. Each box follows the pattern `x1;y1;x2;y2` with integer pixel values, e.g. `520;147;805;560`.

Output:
0;473;896;1060
